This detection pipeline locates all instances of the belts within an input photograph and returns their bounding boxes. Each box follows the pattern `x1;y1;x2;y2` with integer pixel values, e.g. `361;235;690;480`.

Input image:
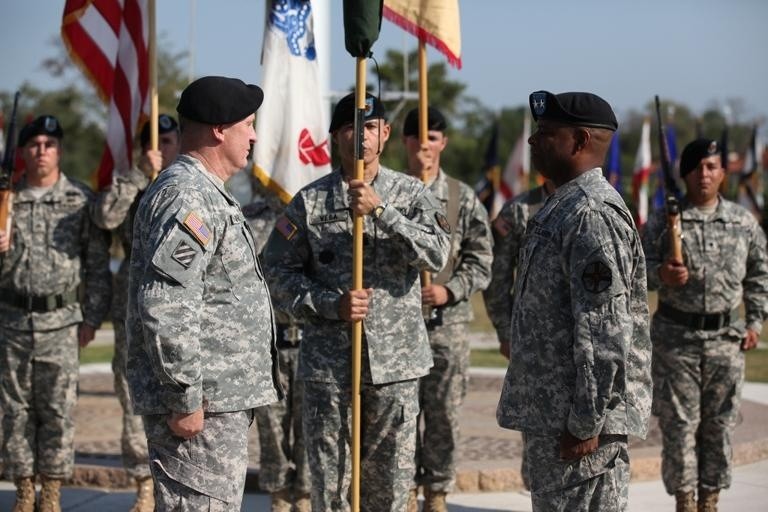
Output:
276;323;307;348
1;283;86;312
657;301;739;329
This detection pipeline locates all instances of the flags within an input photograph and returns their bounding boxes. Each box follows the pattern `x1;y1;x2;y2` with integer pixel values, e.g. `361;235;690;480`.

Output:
381;0;462;67
630;122;650;229
61;0;150;190
654;96;681;211
491;112;531;217
609;134;622;195
251;0;332;205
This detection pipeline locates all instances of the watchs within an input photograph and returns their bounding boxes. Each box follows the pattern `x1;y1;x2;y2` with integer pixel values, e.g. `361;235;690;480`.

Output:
370;202;386;219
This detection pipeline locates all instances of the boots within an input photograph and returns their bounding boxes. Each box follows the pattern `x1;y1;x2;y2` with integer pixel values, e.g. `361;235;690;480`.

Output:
700;488;719;512
11;476;36;512
40;479;62;512
271;491;293;512
294;494;311;512
128;478;154;512
676;490;697;512
425;490;445;511
407;488;419;511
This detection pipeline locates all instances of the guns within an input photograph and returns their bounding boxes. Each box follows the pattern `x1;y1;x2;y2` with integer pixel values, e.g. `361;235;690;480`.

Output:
655;95;685;266
1;91;24;252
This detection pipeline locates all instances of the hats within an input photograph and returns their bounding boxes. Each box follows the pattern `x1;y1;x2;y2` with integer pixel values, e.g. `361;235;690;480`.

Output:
175;76;264;124
530;90;618;132
16;115;64;146
403;108;446;135
329;92;386;132
679;139;724;176
139;114;178;145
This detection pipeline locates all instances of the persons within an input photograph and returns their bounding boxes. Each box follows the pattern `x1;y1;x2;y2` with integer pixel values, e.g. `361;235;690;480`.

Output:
124;74;282;512
92;114;177;512
260;95;450;512
498;90;654;512
486;179;601;488
1;116;112;512
639;139;768;511
386;106;493;512
